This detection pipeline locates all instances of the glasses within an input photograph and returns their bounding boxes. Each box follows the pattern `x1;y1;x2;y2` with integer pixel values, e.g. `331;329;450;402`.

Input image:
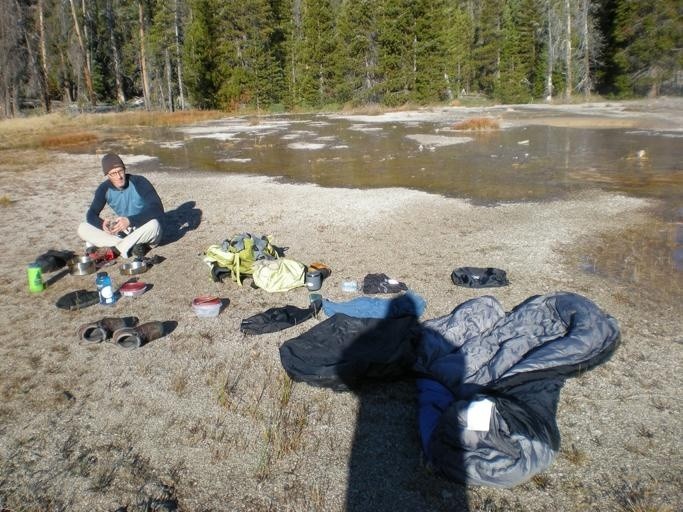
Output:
106;169;123;177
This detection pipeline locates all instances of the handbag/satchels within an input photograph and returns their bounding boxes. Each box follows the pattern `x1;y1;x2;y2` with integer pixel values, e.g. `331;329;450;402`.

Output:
203;231;279;282
56;290;98;311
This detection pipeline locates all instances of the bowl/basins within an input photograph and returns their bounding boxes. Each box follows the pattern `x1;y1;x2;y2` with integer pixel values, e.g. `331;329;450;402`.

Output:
66;256;96;276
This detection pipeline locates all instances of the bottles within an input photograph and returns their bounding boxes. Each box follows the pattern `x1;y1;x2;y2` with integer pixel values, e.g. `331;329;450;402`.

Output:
94;271;114;305
305;271;321;290
26;263;43;292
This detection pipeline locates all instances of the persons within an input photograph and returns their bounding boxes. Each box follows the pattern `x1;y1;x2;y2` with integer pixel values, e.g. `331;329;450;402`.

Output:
77;151;167;269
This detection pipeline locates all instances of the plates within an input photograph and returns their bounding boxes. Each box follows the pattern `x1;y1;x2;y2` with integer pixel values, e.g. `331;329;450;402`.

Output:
118;262;146;275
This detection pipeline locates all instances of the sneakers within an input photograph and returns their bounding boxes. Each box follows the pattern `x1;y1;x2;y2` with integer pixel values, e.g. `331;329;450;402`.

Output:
81;318;128;343
112;321;164;348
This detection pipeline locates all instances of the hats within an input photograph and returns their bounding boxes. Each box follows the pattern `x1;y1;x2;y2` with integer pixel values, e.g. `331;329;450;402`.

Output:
102;153;125;175
